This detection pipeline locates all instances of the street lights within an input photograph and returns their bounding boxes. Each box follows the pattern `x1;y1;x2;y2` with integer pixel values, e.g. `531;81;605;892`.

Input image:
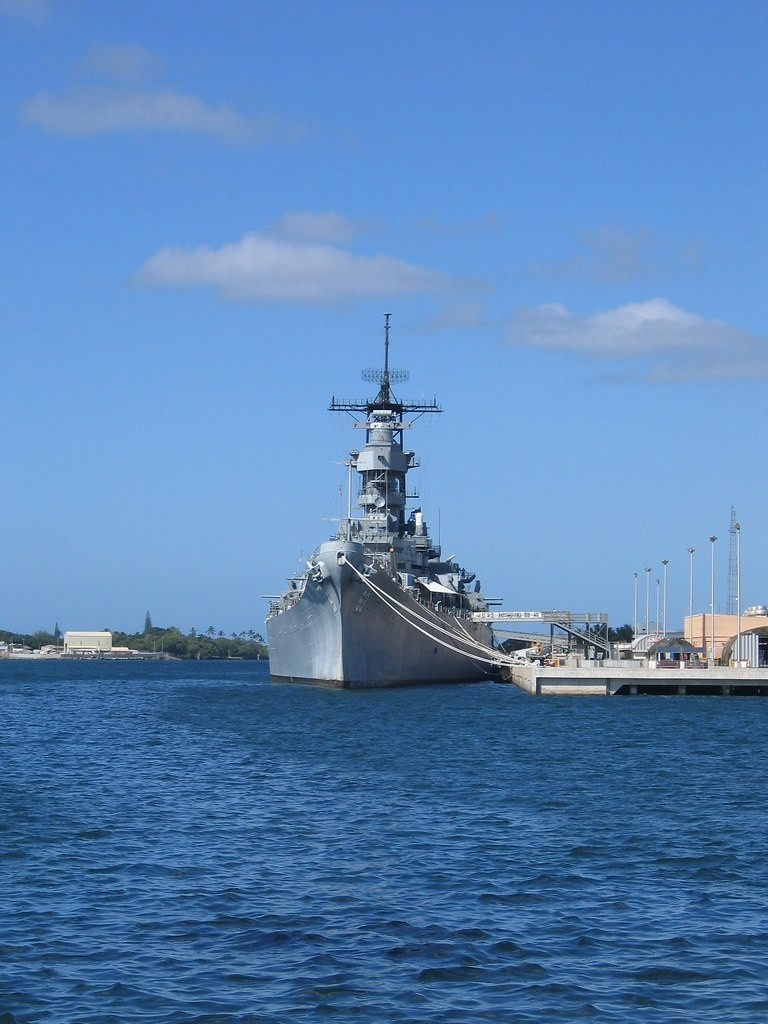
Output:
708;535;717;667
688;546;695;644
634;572;637;640
645;568;651;635
662;558;669;636
656;578;660;635
733;522;741;665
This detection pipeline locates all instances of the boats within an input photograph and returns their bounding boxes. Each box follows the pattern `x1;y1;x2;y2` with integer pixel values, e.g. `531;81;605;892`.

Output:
264;312;494;686
